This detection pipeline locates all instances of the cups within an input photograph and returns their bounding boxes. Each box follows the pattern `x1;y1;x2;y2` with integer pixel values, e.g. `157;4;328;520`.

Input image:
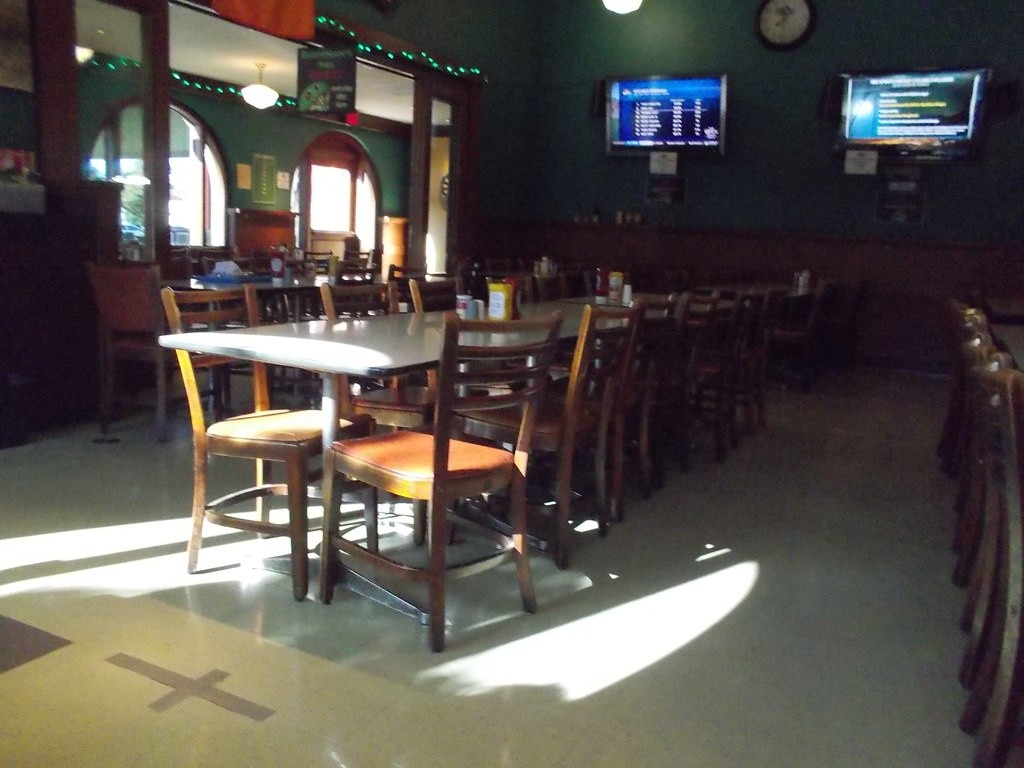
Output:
488;283;513;321
608;271;624;302
329;255;339;275
622;285;632;306
596;266;608;306
455;294;472;320
502;275;522;320
270;251;285;287
474;300;485;321
467;299;479;321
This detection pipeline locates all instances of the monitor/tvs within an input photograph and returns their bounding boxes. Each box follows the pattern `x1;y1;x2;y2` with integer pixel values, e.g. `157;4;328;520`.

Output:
833;65;993;165
603;73;727;156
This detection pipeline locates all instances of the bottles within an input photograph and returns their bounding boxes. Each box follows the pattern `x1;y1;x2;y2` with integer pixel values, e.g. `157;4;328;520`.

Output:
304;254;316;282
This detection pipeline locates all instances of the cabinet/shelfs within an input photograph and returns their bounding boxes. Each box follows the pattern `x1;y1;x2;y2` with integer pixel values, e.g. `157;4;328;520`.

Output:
0;182;52;448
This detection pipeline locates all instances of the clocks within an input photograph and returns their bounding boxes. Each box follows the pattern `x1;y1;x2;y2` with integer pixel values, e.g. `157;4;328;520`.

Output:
756;0;815;52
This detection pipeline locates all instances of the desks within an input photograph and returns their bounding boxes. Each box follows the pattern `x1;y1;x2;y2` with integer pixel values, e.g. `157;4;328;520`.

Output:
565;295;749;326
159;301;667;604
695;280;824;301
162;276;376;411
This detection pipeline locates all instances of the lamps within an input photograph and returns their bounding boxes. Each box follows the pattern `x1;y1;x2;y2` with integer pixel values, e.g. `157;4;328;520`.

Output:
239;62;279;110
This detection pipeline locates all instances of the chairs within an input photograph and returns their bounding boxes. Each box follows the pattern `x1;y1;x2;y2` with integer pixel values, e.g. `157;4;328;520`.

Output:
938;297;1024;768
83;241;867;650
